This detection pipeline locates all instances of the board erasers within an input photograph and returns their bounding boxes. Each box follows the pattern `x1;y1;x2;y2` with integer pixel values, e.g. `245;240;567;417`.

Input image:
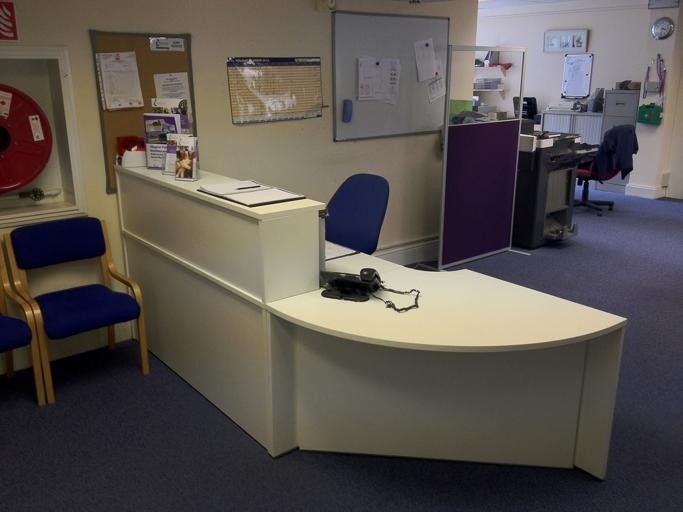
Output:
343;99;352;122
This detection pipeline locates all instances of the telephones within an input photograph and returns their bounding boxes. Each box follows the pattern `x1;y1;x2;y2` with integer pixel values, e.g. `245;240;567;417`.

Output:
320;268;382;295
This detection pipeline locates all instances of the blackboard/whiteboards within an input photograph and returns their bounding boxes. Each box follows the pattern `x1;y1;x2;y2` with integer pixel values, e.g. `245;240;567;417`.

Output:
333;11;448;140
561;54;594;98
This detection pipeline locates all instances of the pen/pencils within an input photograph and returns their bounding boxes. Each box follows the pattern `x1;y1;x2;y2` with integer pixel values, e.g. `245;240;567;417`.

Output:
237;186;261;189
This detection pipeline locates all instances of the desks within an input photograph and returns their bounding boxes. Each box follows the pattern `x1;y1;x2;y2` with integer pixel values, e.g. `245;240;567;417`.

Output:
109;156;629;484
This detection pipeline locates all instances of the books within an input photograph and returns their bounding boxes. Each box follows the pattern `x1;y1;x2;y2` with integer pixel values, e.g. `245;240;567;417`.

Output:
195;179;305;208
95;49;145;113
142;110;182;169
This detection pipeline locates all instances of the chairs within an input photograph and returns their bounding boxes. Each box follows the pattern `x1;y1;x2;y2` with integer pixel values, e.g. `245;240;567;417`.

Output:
324;173;389;255
0;241;47;408
575;124;636;216
5;216;151;404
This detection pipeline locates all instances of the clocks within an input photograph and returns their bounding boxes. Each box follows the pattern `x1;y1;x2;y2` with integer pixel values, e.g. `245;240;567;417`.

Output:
651;17;675;40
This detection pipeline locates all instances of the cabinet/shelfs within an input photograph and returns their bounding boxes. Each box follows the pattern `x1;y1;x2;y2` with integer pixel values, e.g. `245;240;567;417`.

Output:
598;89;640;185
540;110;603;190
